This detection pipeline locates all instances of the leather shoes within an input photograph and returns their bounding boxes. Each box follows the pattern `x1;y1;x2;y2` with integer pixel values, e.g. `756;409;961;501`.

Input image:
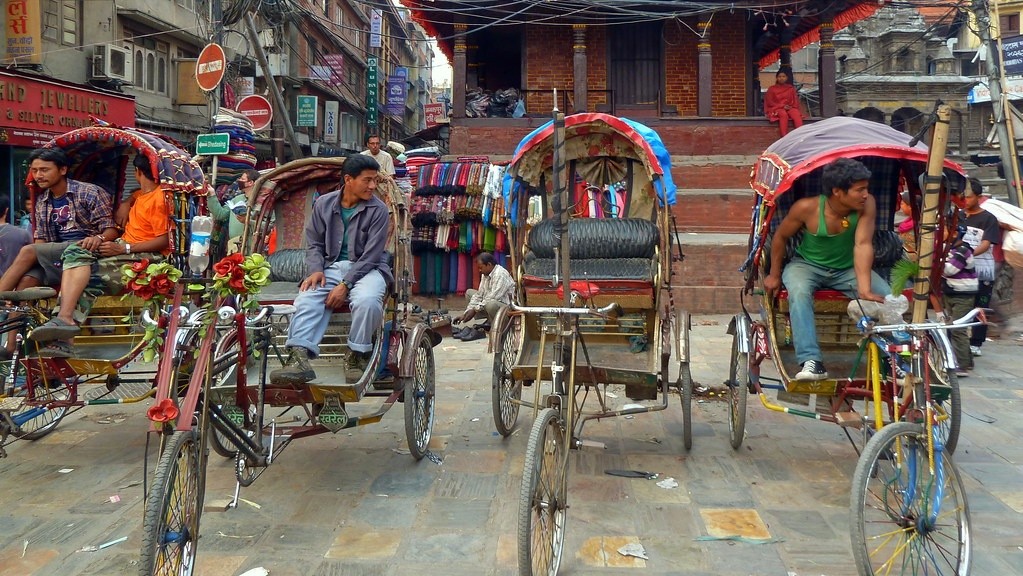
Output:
460;328;486;341
453;325;473;339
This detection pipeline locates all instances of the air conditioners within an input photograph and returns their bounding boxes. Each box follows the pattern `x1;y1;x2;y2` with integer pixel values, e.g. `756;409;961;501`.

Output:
93;43;134;85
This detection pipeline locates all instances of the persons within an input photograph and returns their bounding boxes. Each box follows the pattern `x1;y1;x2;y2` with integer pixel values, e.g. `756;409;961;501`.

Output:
270;154;395;385
360;133;396;177
764;68;808;136
891;178;1002;377
208;169;275;335
765;157;906;385
452;252;516;331
0;149;177;396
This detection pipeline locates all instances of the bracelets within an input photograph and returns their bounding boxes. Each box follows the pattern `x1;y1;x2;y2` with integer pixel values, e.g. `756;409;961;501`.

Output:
96;234;105;242
126;243;130;254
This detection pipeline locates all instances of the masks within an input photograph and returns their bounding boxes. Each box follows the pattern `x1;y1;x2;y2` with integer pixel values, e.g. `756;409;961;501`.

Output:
237;181;251;190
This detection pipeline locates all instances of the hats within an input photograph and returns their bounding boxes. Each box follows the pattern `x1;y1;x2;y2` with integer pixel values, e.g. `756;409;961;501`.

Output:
899;190;922;207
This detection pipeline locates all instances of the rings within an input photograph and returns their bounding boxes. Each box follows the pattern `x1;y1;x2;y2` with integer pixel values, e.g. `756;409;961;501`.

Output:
306;281;307;282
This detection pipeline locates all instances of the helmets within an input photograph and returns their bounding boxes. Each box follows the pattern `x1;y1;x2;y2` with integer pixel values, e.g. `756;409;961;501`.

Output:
934;210;969;235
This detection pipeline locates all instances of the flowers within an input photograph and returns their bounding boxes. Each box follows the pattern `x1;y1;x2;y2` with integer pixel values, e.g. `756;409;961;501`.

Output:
122;259;183;363
194;253;272;360
147;399;180;429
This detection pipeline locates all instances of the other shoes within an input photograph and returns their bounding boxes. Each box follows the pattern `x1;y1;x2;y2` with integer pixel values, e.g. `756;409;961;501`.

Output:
0;345;13;368
343;347;364;384
269;347;316;386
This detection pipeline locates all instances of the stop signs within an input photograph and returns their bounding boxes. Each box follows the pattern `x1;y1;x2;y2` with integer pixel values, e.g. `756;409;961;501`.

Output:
195;42;226;91
236;94;274;131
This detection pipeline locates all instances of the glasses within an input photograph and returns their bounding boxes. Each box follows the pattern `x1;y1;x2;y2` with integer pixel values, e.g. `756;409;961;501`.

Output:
367;142;380;145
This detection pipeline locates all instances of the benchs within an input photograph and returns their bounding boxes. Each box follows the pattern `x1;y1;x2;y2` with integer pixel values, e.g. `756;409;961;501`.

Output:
522;218;659;309
252;249;394;334
778;232;914;316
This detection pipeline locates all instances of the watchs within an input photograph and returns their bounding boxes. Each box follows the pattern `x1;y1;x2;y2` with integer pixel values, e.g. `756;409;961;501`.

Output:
342;281;352;290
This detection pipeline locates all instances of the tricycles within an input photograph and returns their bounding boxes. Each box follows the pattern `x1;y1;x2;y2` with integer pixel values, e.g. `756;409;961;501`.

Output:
0;114;212;459
721;102;1000;576
484;85;702;576
135;154;454;576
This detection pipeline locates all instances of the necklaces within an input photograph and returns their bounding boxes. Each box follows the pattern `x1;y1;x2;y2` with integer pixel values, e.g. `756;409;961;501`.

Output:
829;203;849;227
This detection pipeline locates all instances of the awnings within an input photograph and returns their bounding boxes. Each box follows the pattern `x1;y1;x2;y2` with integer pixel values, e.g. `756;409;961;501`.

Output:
400;124;449;155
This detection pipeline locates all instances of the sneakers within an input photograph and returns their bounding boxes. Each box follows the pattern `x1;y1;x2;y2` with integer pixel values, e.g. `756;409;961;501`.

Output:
943;365;969;377
795;360;827;382
970;345;982;356
966;361;975;370
885;355;907;386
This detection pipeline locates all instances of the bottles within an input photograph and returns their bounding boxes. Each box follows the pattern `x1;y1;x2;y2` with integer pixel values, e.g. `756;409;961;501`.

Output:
188;216;213;279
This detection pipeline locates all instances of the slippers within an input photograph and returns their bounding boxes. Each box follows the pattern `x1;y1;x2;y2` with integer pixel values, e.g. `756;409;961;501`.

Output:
29;317;82;342
38;340;80;359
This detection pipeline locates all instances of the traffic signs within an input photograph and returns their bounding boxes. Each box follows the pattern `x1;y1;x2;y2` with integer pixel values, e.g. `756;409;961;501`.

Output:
196;132;230;156
